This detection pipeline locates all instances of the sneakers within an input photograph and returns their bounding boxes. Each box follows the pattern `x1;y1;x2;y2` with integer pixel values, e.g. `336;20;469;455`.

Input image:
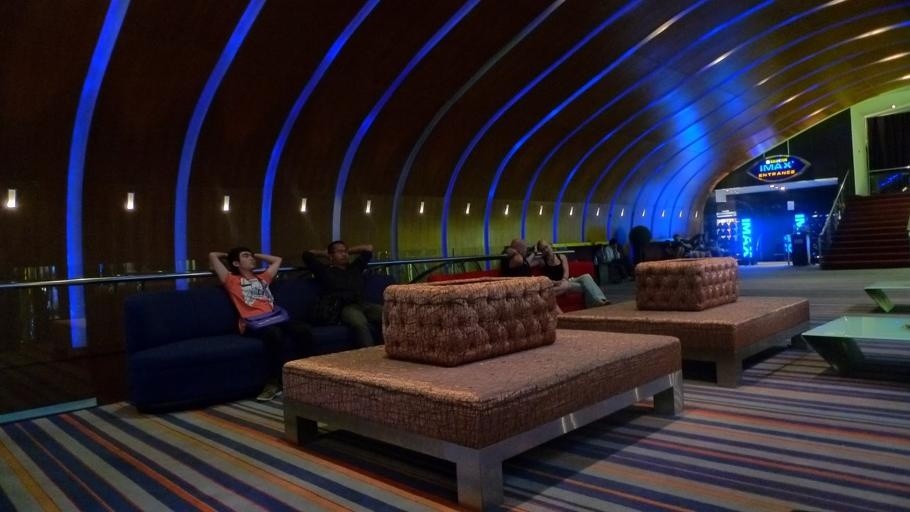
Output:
256;383;283;401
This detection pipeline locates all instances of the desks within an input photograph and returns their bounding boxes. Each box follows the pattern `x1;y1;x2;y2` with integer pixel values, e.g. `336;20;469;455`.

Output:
801;316;910;374
864;281;910;314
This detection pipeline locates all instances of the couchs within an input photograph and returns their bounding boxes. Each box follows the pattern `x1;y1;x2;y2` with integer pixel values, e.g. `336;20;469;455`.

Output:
125;275;398;415
429;260;596;311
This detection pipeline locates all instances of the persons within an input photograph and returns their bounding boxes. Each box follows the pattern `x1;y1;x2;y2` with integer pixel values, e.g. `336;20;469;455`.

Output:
208;244;333;401
526;239;611;308
302;240;386;349
593;232;723;282
500;239;565;315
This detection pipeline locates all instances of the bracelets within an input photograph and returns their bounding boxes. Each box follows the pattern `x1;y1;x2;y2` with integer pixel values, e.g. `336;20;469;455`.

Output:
223;252;230;259
348;247;352;255
533;251;537;256
321;249;326;257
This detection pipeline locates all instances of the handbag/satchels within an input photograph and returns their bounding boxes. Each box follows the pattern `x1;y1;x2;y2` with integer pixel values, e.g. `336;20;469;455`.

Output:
243;305;290;335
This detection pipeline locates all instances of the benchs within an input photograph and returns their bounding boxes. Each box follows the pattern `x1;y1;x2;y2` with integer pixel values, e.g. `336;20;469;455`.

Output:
283;328;686;511
557;297;811;386
